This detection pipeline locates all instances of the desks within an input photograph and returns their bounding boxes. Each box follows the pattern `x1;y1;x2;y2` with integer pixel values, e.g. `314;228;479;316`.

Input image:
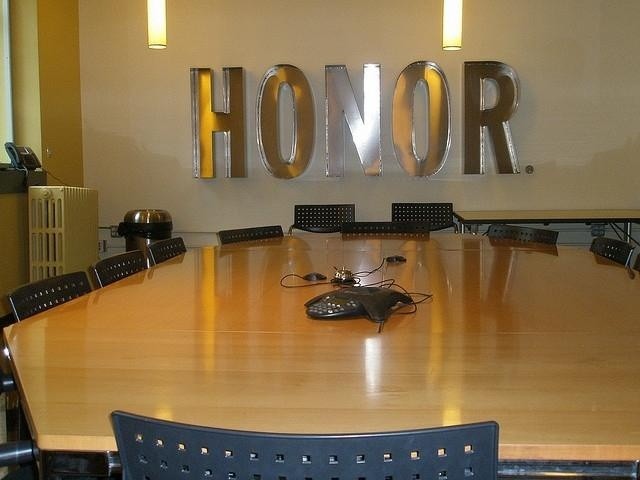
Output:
452;209;640;243
4;232;639;478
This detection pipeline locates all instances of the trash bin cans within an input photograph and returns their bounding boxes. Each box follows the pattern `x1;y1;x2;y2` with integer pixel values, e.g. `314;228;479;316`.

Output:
124;209;173;252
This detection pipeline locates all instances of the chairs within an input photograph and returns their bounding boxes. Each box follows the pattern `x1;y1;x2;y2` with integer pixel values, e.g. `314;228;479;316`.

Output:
288;204;356;233
109;410;500;479
485;223;560;244
8;272;93;322
146;236;188;265
217;225;285;246
93;250;150;288
392;204;458;232
340;222;432;233
589;236;639;267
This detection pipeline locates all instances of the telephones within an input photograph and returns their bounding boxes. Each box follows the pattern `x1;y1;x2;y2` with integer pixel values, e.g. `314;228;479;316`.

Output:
4;142;42;170
304;287;414;323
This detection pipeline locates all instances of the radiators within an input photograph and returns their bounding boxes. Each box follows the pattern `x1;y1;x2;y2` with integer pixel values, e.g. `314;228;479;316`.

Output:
28;186;99;283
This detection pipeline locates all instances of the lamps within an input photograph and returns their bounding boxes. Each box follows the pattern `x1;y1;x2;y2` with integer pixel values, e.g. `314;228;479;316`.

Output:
442;0;463;51
147;0;167;50
